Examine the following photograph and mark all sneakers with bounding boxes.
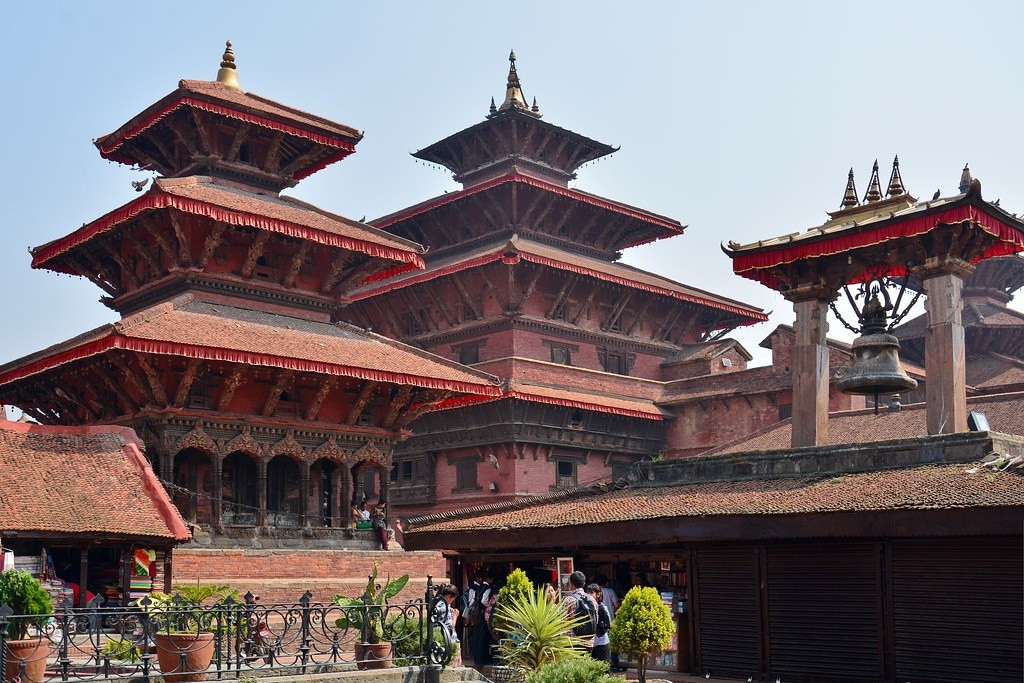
[612,666,627,672]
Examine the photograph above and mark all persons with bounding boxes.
[349,500,363,529]
[431,569,627,677]
[370,499,389,550]
[57,579,99,633]
[356,502,373,528]
[322,498,329,523]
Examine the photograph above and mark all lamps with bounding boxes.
[967,410,990,432]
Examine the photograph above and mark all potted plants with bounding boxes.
[127,591,249,683]
[332,560,412,671]
[0,566,55,682]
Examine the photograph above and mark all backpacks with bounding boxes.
[570,593,597,638]
[459,584,507,641]
